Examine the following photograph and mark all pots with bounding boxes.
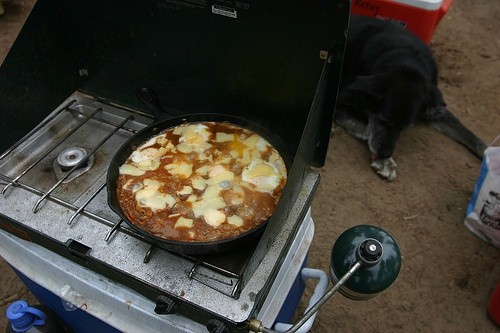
[106,114,290,253]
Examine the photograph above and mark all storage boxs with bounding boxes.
[351,0,452,47]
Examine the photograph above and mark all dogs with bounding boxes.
[333,12,488,182]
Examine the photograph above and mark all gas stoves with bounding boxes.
[0,91,322,333]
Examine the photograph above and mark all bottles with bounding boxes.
[5,300,46,333]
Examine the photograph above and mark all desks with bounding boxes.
[0,205,329,333]
